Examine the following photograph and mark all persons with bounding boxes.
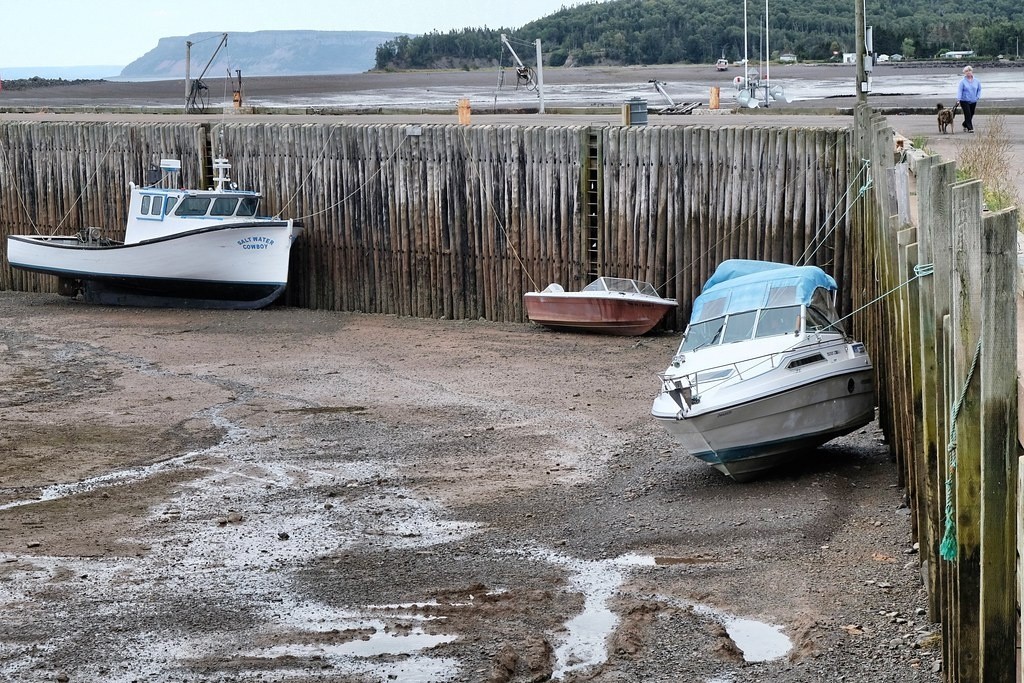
[957,66,981,132]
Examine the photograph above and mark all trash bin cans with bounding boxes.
[625,96,648,125]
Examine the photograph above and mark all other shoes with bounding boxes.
[968,130,974,132]
[962,123,967,132]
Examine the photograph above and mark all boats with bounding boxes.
[524,277,678,336]
[651,258,875,485]
[7,71,304,310]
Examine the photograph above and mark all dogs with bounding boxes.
[937,103,954,134]
